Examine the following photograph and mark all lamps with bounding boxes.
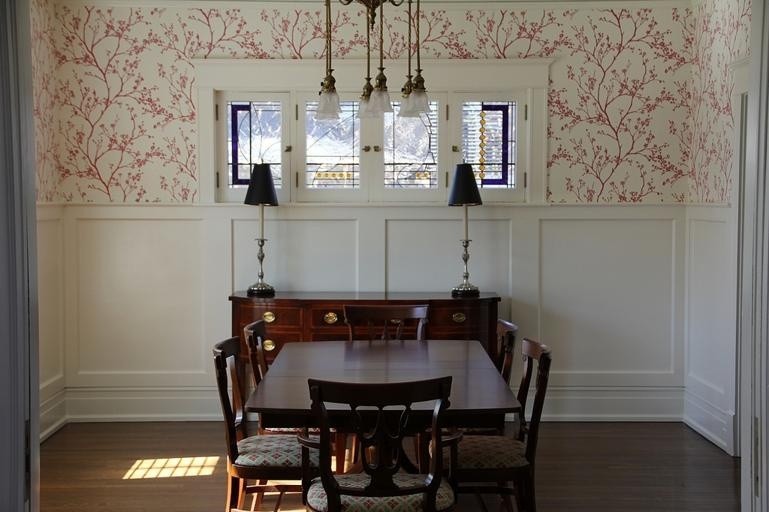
[448,162,483,297]
[315,0,432,122]
[243,163,279,296]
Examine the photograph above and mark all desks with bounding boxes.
[228,291,502,441]
[240,338,524,512]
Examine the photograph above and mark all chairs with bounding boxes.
[434,317,523,463]
[213,335,331,511]
[242,317,348,476]
[339,303,434,476]
[441,334,552,511]
[303,374,457,510]
